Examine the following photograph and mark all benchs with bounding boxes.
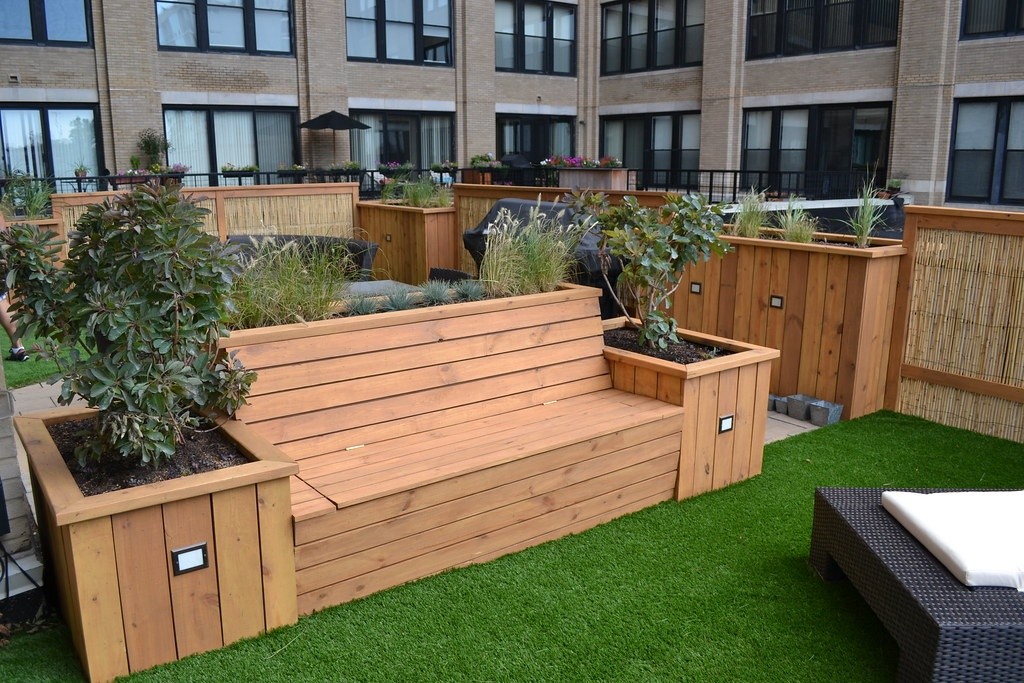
[208,289,684,620]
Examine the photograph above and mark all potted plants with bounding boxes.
[594,191,783,504]
[1,167,299,679]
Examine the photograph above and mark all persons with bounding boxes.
[0,248,30,361]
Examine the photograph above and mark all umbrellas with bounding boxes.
[295,109,372,169]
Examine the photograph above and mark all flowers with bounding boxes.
[322,152,623,170]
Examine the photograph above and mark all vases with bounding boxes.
[768,394,777,411]
[774,396,788,414]
[810,399,844,429]
[787,393,817,420]
